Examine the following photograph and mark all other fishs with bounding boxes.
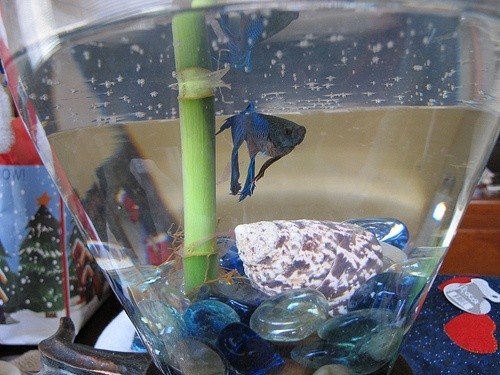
[215,103,307,202]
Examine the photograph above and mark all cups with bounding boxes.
[0,0,500,375]
